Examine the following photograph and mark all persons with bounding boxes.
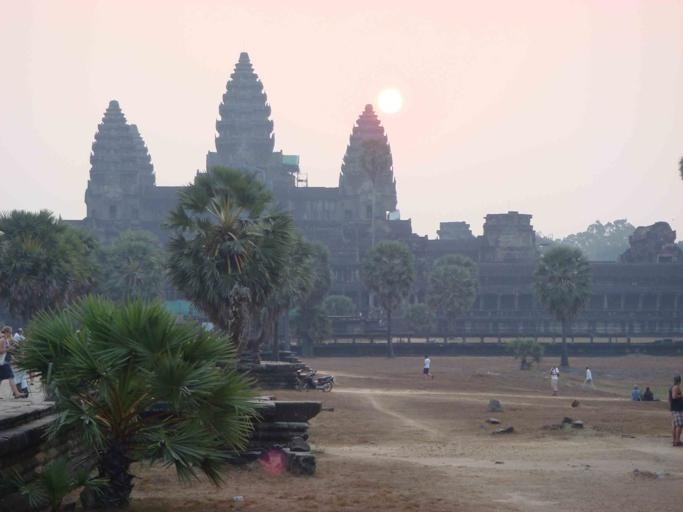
[668,374,683,446]
[631,384,640,401]
[0,326,28,398]
[582,366,593,392]
[423,354,434,381]
[642,386,654,401]
[550,364,559,396]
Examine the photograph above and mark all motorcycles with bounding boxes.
[300,367,335,393]
[293,368,308,391]
